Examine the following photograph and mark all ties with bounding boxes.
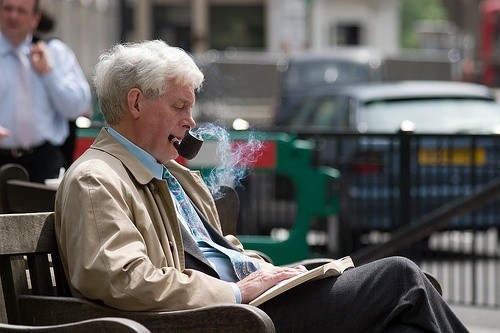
[14,47,33,149]
[163,166,258,280]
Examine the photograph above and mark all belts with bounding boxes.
[0,142,51,158]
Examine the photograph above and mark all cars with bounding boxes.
[287,83,500,261]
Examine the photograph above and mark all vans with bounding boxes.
[272,48,387,139]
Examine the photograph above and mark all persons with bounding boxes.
[54,37,471,333]
[0,0,92,269]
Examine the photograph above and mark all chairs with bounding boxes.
[0,164,443,333]
